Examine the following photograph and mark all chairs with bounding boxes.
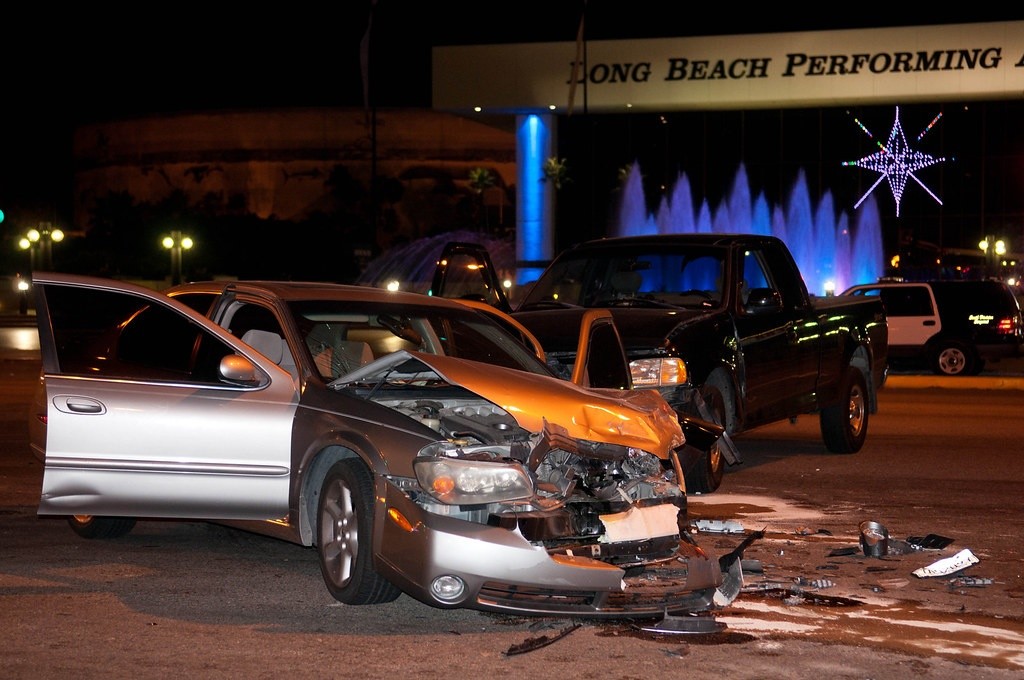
[330,341,375,378]
[715,275,745,302]
[610,272,642,298]
[235,330,283,365]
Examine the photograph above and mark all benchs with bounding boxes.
[278,338,314,381]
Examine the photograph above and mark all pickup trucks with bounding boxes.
[429,232,893,494]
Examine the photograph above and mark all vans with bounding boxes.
[828,274,1024,378]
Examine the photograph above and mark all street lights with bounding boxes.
[19,218,66,273]
[977,235,1009,277]
[162,229,197,290]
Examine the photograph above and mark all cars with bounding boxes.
[26,271,726,621]
